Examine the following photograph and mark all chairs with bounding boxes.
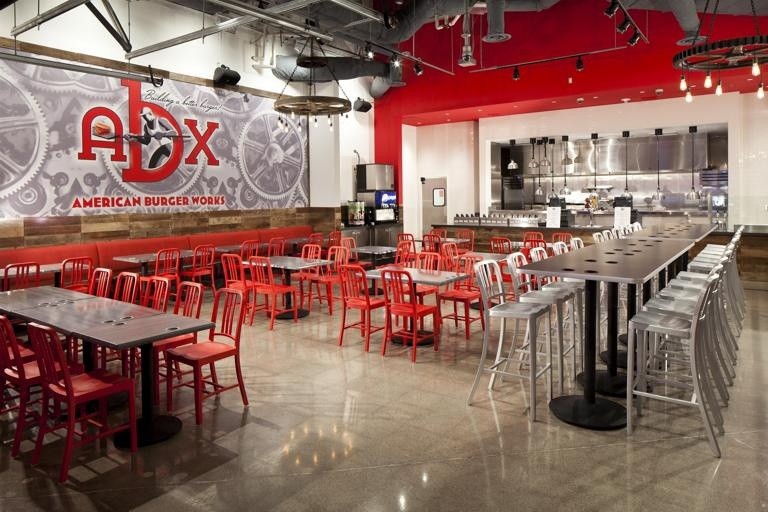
[336,229,573,363]
[113,230,353,331]
[1,257,249,487]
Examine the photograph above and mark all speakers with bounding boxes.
[214,67,240,86]
[354,100,371,112]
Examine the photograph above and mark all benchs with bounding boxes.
[1,225,317,286]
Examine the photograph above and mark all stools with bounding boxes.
[469,221,748,460]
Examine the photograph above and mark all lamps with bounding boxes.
[510,53,585,82]
[507,125,700,200]
[275,35,351,132]
[365,39,424,76]
[603,0,647,49]
[671,0,768,105]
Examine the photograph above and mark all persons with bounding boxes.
[124,104,178,169]
